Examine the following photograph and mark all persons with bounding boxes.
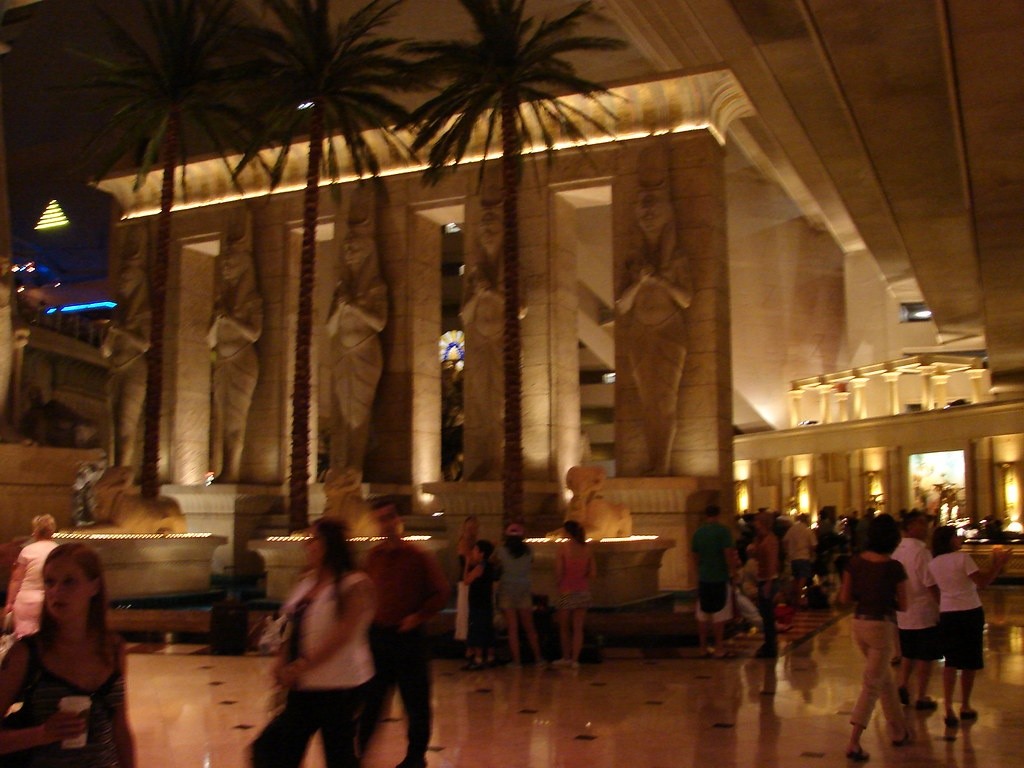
[924,525,1013,727]
[615,146,695,479]
[327,187,386,493]
[355,494,452,768]
[737,506,940,710]
[97,231,153,467]
[838,513,915,761]
[461,194,529,481]
[203,209,263,486]
[0,514,137,768]
[454,515,597,673]
[691,504,741,660]
[251,518,377,768]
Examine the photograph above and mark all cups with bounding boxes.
[58,695,92,749]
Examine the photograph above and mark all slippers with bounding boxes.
[893,730,909,745]
[847,746,870,760]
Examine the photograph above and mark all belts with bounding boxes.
[854,614,899,623]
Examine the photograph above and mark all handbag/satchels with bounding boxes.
[0,612,15,662]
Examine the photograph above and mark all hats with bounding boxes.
[506,524,524,537]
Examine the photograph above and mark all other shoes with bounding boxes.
[552,658,580,669]
[945,717,958,727]
[777,625,792,632]
[461,659,495,671]
[756,644,776,657]
[899,686,908,704]
[916,699,937,709]
[962,709,975,719]
[891,657,899,663]
